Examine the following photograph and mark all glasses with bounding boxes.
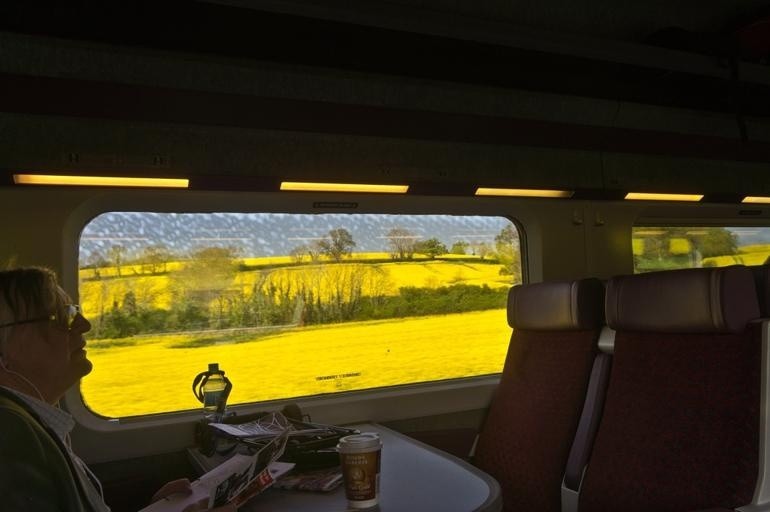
[0,304,82,332]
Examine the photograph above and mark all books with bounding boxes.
[139,453,296,511]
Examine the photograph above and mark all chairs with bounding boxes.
[561,266,769,512]
[466,276,604,512]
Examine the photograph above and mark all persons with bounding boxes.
[0,265,194,512]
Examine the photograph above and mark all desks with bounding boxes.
[187,421,504,512]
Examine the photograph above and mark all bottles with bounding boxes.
[201,362,229,432]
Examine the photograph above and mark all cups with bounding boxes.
[335,433,384,509]
